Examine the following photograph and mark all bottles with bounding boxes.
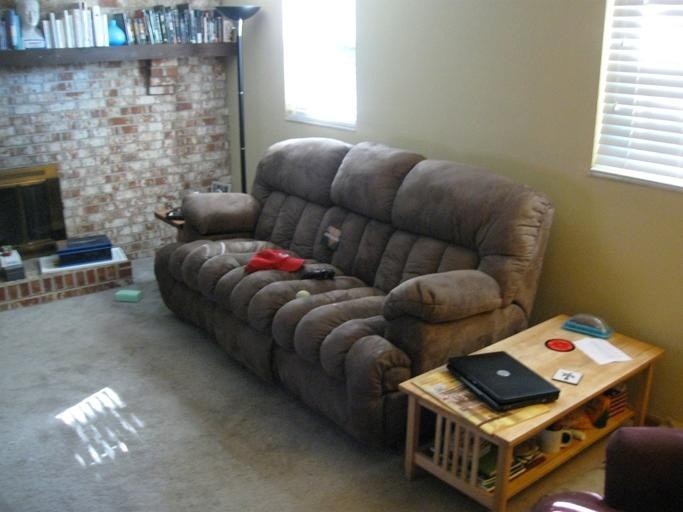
[107,19,126,45]
[5,7,21,52]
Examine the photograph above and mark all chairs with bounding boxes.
[533,426,683,512]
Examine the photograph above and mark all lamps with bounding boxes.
[216,5,261,193]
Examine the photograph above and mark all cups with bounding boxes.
[542,426,573,454]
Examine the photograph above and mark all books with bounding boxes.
[42,0,237,49]
[604,388,629,417]
[430,426,548,493]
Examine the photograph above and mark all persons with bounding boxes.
[16,0,45,49]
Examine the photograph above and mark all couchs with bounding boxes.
[153,137,556,455]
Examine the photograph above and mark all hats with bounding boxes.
[243,248,306,272]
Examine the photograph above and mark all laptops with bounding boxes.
[447,351,563,410]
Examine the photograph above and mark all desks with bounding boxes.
[153,205,184,228]
[398,313,667,510]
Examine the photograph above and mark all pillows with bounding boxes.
[382,268,502,325]
[179,192,261,236]
